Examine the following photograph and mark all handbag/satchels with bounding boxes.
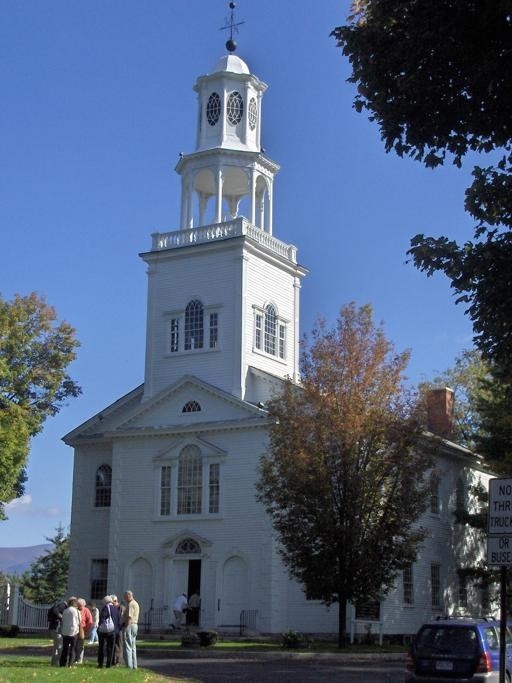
[97,604,114,633]
[79,624,84,639]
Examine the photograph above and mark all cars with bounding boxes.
[405,616,512,683]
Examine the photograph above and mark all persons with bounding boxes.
[173,590,201,628]
[47,588,139,670]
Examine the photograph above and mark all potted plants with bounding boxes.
[182,630,218,650]
[280,629,313,648]
